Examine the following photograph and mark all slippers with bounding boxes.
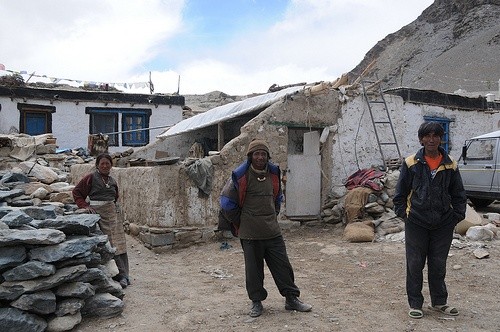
[409,308,423,318]
[429,304,459,315]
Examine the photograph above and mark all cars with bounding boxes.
[456,130,500,210]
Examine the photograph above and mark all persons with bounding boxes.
[218,140,313,317]
[393,121,467,319]
[72,154,131,286]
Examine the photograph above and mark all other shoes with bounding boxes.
[121,277,128,287]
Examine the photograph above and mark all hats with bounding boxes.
[247,140,269,153]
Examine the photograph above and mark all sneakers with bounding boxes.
[250,300,263,317]
[285,296,313,311]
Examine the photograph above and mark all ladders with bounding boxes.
[359,72,404,173]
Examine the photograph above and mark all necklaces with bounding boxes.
[102,177,110,188]
[257,177,265,181]
